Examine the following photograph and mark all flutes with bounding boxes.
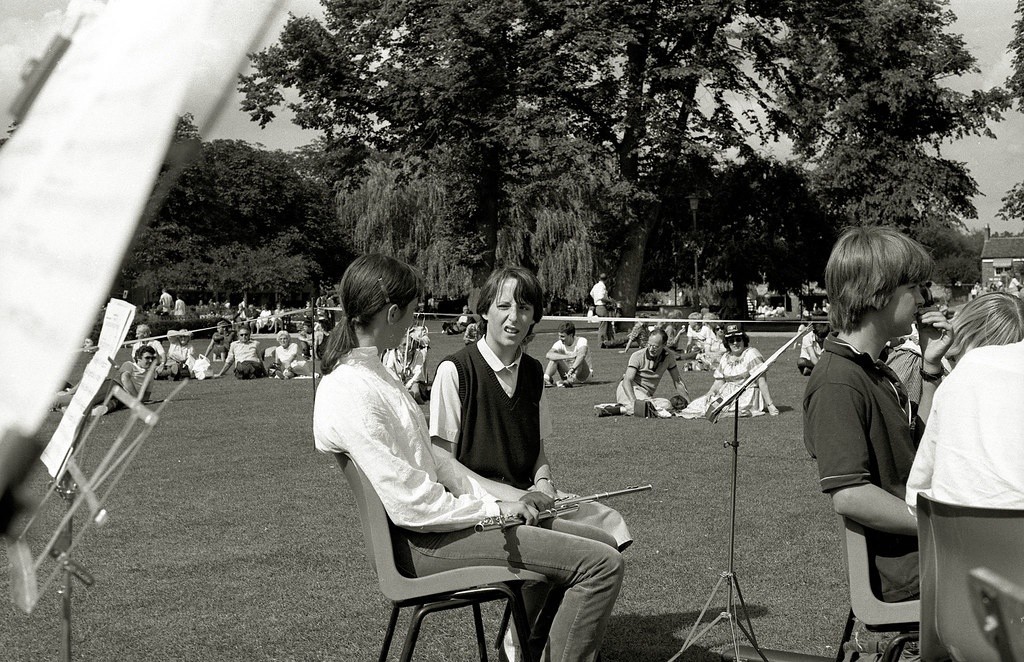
[473,483,653,532]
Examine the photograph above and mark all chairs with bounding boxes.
[835,491,1024,662]
[334,452,548,662]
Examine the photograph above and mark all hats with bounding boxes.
[723,323,743,338]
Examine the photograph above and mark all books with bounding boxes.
[40,298,137,480]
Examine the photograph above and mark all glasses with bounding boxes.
[646,344,664,350]
[144,356,157,362]
[240,333,249,337]
[727,338,743,343]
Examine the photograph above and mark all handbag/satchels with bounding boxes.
[634,399,658,419]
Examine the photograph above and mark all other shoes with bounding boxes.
[544,373,556,388]
[90,405,108,417]
[802,367,812,376]
[236,360,255,379]
[593,404,623,417]
[167,364,180,382]
[561,379,574,388]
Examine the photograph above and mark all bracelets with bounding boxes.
[536,477,554,487]
[918,365,944,382]
[768,401,773,406]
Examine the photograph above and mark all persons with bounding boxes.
[677,323,779,419]
[920,281,935,308]
[747,297,787,318]
[204,317,329,380]
[797,324,830,376]
[82,334,99,353]
[802,226,954,603]
[971,273,1024,300]
[594,329,691,418]
[49,345,156,416]
[132,324,194,380]
[314,254,632,662]
[884,292,1024,402]
[906,337,1024,526]
[603,310,725,371]
[159,287,287,335]
